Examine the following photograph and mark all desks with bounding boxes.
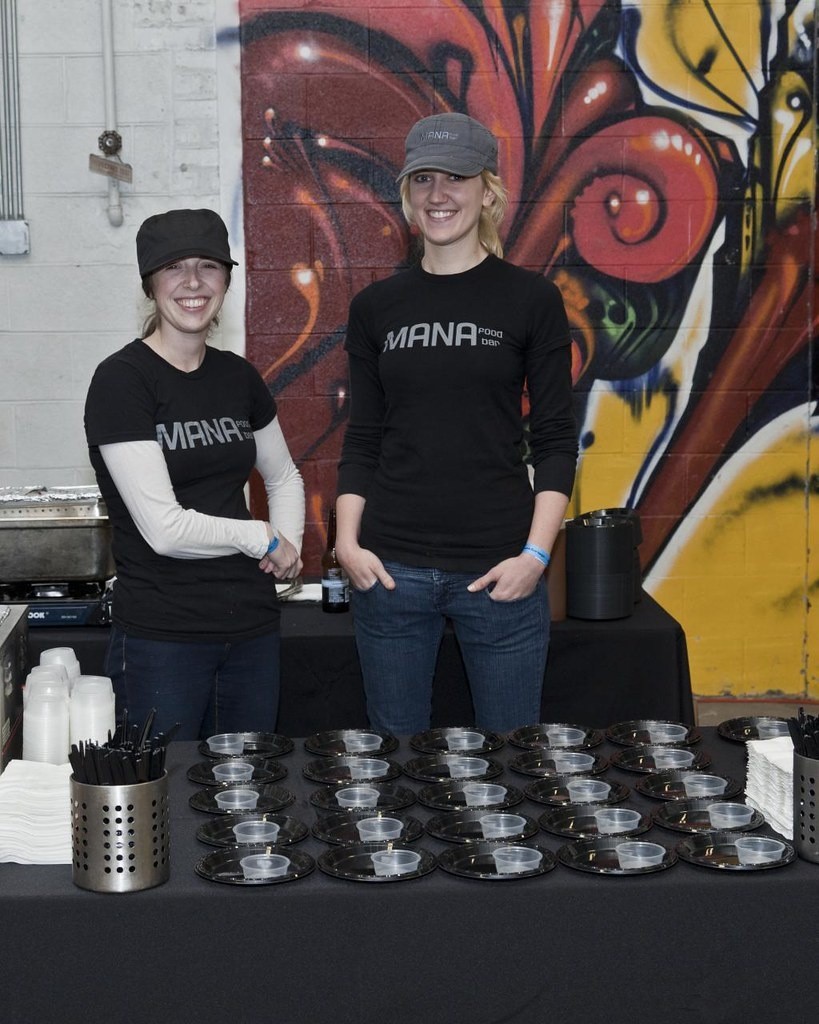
[26,575,698,742]
[1,724,819,1024]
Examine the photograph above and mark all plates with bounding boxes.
[565,507,643,621]
[186,715,797,887]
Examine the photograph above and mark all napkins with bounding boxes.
[1,759,77,865]
[743,736,795,841]
[275,583,324,602]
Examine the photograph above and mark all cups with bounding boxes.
[22,648,116,766]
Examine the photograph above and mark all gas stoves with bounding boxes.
[0,576,107,604]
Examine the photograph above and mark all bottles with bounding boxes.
[322,510,350,613]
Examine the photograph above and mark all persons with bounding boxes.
[84,208,305,741]
[333,112,579,735]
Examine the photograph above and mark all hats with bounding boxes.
[395,112,497,183]
[136,209,239,277]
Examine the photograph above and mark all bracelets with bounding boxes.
[267,525,279,554]
[522,543,550,567]
